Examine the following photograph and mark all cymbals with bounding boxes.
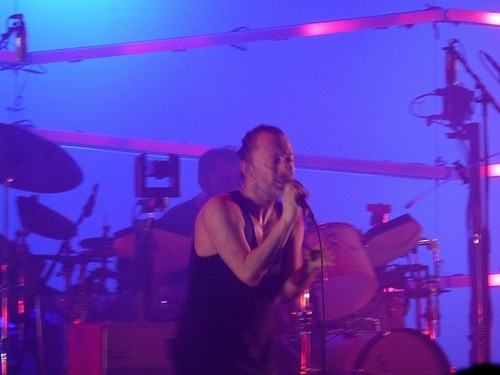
[0,120,83,194]
[17,195,78,240]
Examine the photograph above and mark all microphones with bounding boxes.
[445,52,456,85]
[295,195,314,220]
[84,184,98,216]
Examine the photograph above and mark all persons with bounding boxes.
[153,147,241,239]
[174,124,337,375]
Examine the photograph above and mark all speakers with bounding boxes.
[65,321,179,375]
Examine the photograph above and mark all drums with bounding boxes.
[312,221,379,320]
[311,326,453,375]
